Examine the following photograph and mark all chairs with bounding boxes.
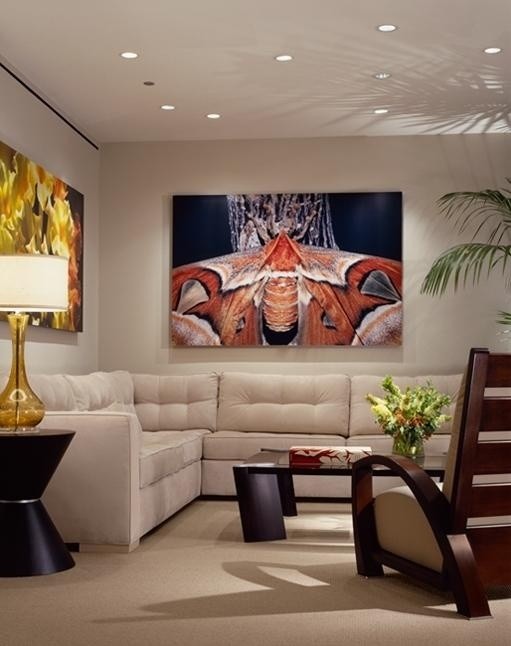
[350,348,511,620]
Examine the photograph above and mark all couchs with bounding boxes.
[201,371,511,500]
[0,369,218,553]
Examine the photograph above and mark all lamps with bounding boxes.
[0,253,70,433]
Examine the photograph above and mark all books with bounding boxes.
[288,446,373,467]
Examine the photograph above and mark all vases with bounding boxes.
[390,434,425,468]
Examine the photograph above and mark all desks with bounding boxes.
[0,429,75,577]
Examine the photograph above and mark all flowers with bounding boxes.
[365,375,453,457]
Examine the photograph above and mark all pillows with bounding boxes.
[347,373,466,435]
[218,372,349,437]
[63,370,143,435]
[131,371,219,432]
[1,374,78,415]
